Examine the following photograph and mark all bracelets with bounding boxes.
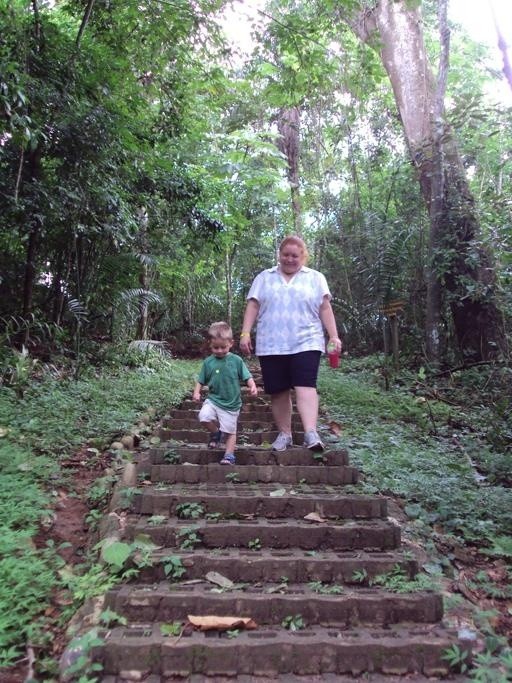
[239,332,250,338]
[330,335,338,338]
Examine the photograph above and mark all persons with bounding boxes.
[193,321,258,466]
[239,235,342,452]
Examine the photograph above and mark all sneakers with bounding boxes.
[304,431,326,450]
[271,432,293,451]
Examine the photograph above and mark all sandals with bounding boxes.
[208,431,221,448]
[220,453,236,465]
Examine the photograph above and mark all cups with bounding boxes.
[326,342,341,368]
